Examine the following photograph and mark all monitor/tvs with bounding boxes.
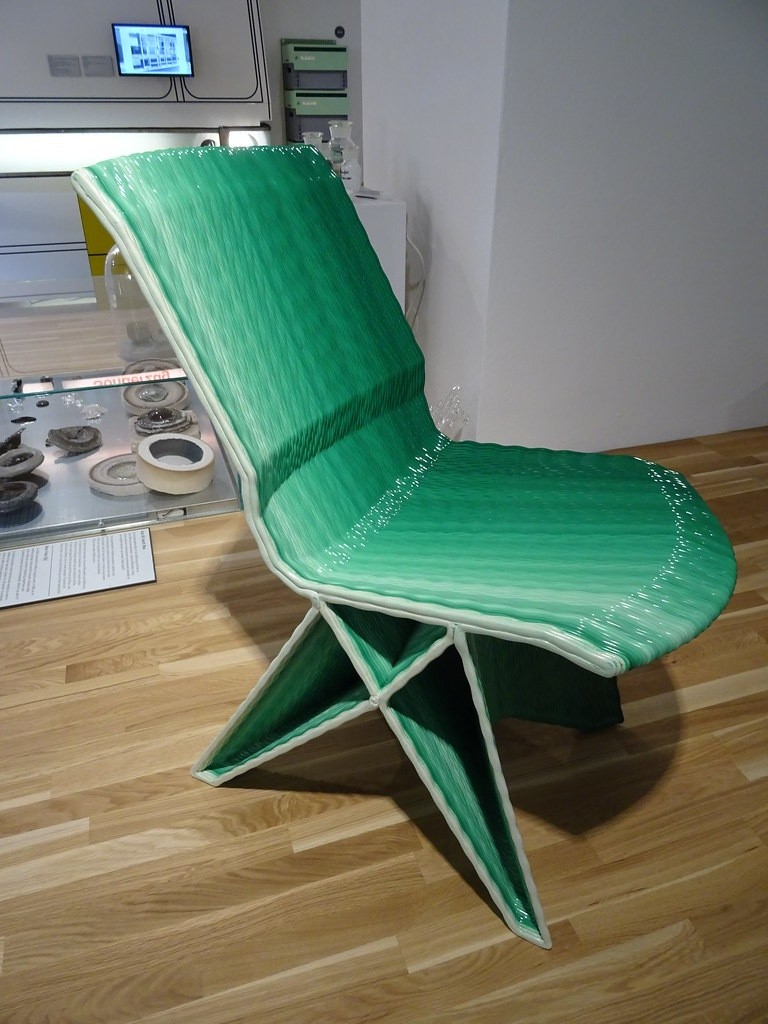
[112,23,194,78]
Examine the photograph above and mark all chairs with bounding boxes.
[72,143,737,951]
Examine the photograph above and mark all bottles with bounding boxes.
[301,130,328,162]
[327,119,363,193]
[104,243,174,361]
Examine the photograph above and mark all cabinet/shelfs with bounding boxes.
[0,126,284,549]
